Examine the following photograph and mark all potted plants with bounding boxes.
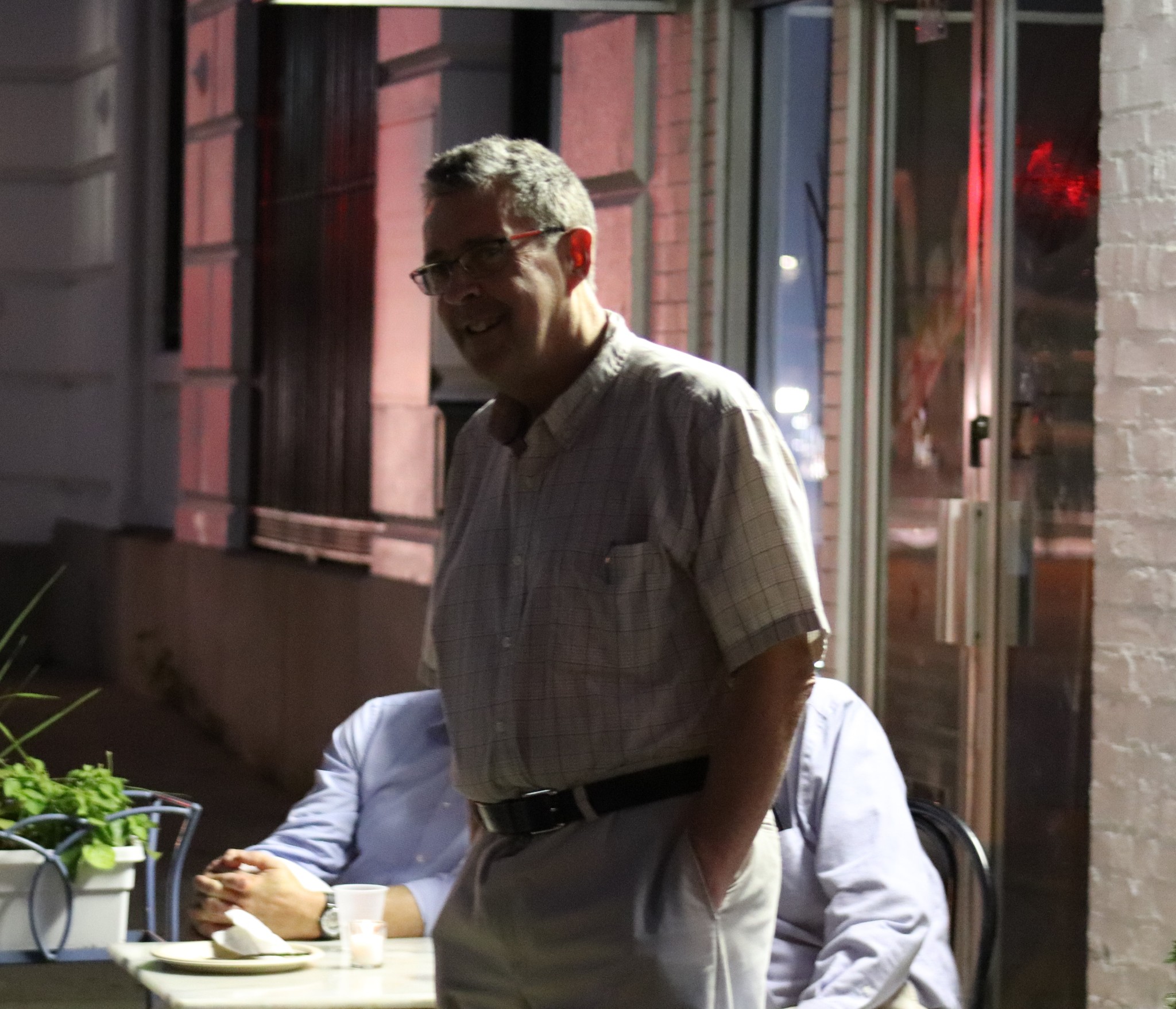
[0,757,165,950]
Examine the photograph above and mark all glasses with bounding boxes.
[409,226,570,296]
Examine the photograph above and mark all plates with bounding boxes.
[151,941,324,973]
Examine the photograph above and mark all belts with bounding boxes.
[469,755,708,834]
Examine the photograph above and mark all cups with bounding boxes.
[332,884,390,950]
[351,920,388,969]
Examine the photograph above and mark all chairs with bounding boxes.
[907,799,1001,1009]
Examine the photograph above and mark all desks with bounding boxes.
[101,920,439,1009]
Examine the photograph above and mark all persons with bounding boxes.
[771,678,967,1009]
[409,134,831,1009]
[191,690,468,942]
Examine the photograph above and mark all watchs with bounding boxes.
[320,890,348,942]
